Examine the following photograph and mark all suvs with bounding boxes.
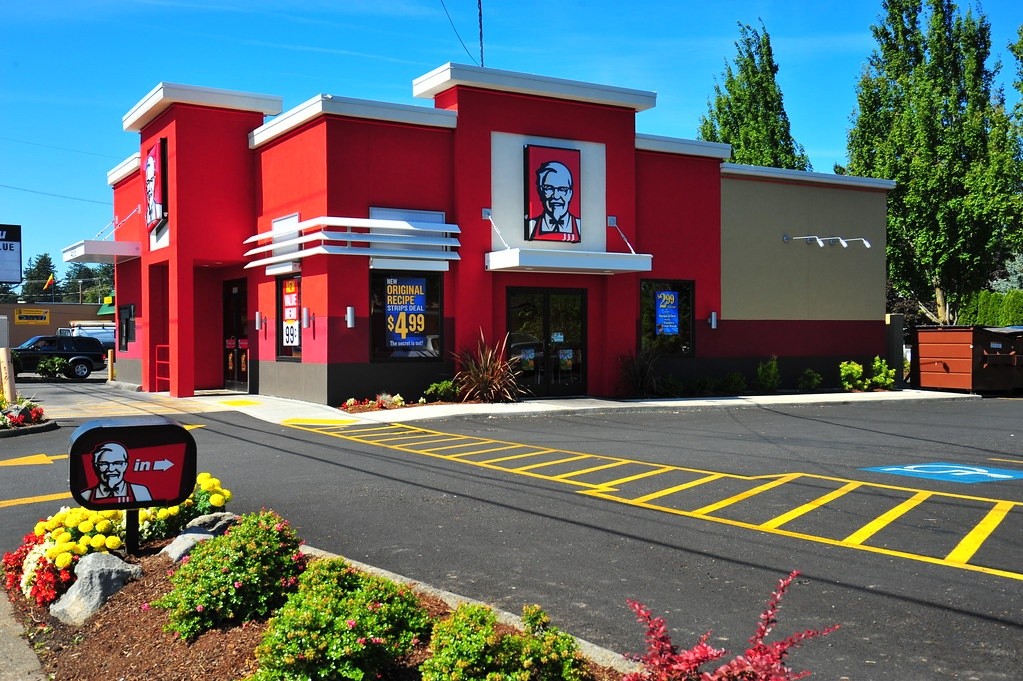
[56,320,116,361]
[10,335,107,379]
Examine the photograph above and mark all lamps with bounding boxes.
[807,237,848,249]
[303,308,315,328]
[255,312,266,330]
[782,235,823,247]
[830,237,871,249]
[346,306,356,329]
